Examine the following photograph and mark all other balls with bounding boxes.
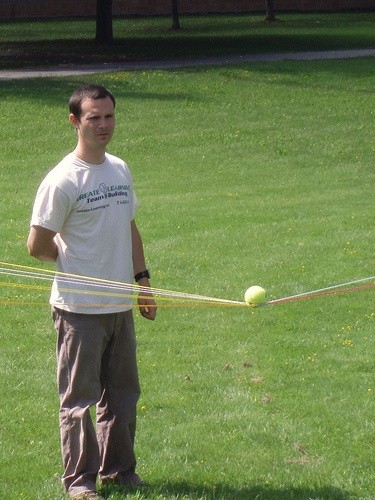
[246,285,266,305]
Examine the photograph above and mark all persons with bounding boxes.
[25,83,159,500]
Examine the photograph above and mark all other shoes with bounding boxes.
[73,490,106,500]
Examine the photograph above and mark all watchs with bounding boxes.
[132,268,152,283]
